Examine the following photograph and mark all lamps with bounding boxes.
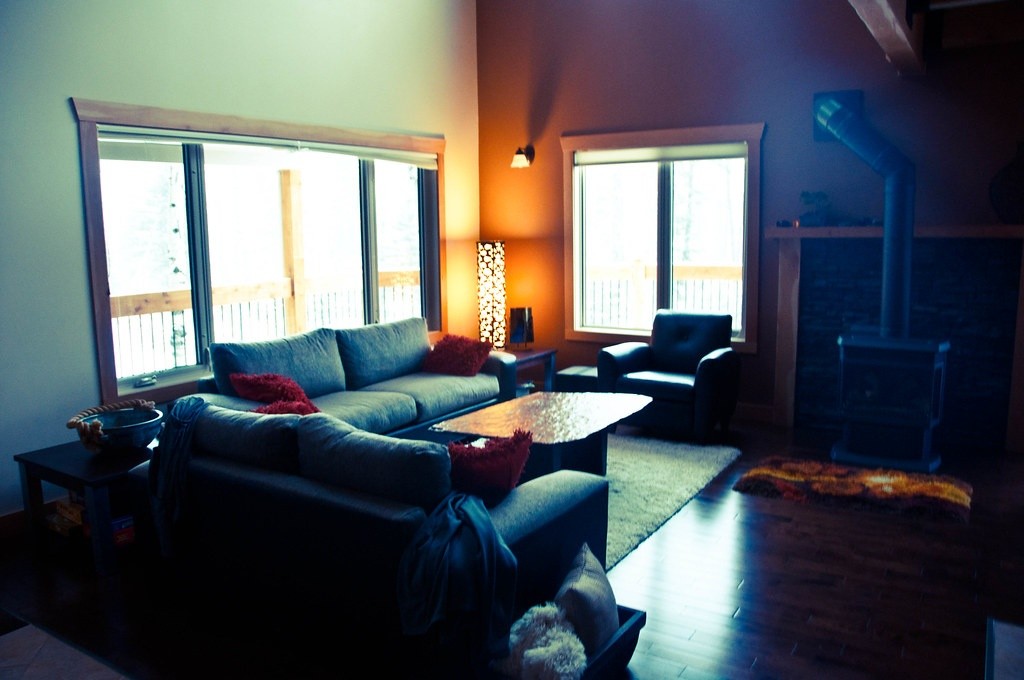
[511,146,535,168]
[509,307,534,351]
[477,239,508,351]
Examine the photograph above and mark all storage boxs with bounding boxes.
[488,605,646,680]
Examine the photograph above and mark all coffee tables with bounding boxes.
[426,391,653,487]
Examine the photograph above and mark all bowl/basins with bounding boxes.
[78,408,164,459]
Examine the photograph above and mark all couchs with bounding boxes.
[166,317,517,447]
[597,309,738,447]
[149,395,609,680]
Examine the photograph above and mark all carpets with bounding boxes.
[464,433,740,571]
[730,447,973,525]
[0,603,143,680]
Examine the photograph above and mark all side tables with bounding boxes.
[14,439,177,635]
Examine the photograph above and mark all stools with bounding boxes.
[555,365,598,392]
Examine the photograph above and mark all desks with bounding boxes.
[508,349,558,398]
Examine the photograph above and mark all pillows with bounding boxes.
[420,333,493,377]
[552,542,620,663]
[250,401,318,416]
[448,428,533,507]
[228,372,321,412]
